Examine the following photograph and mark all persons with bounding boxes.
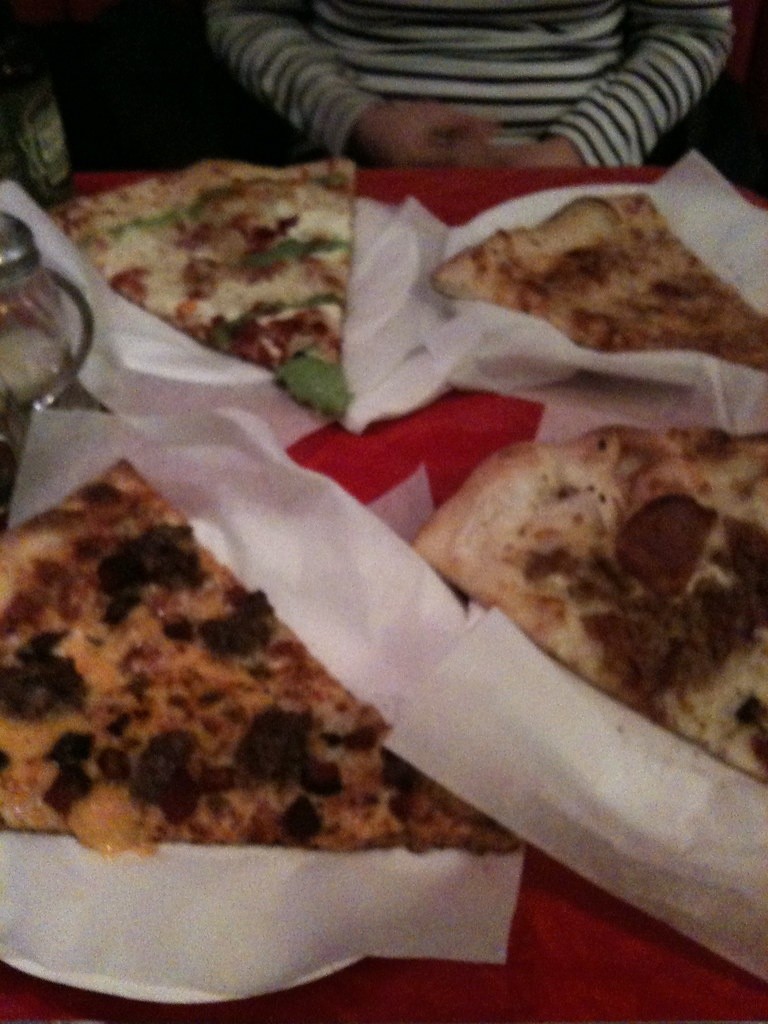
[201,1,742,173]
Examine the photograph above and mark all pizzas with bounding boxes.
[0,154,767,855]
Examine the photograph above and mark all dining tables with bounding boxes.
[1,168,766,1021]
